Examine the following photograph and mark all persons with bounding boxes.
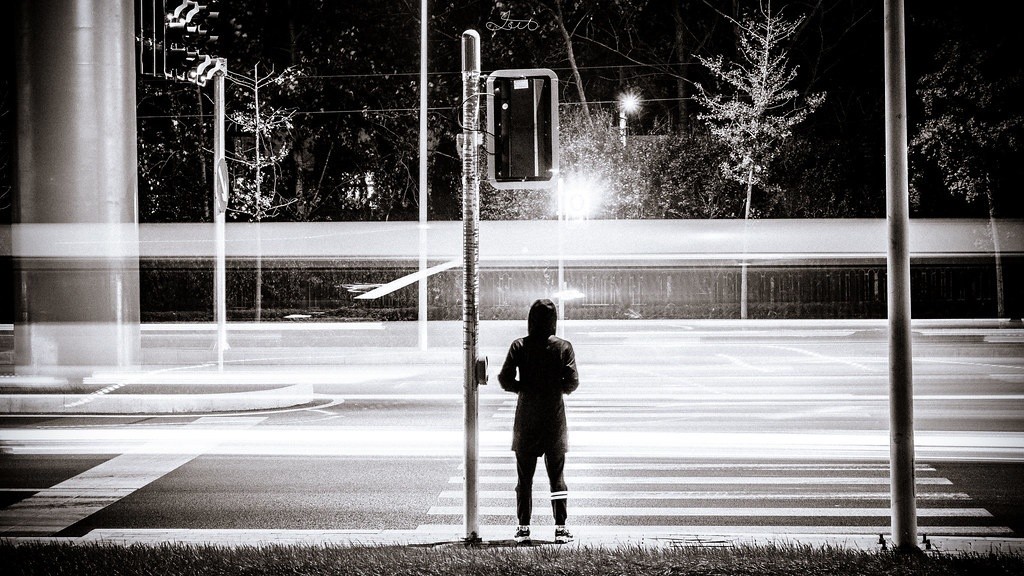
[497,299,580,544]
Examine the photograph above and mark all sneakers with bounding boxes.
[513,527,530,542]
[555,527,574,546]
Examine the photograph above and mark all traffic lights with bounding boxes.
[167,47,222,87]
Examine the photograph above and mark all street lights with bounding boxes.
[558,178,594,320]
[619,92,638,150]
